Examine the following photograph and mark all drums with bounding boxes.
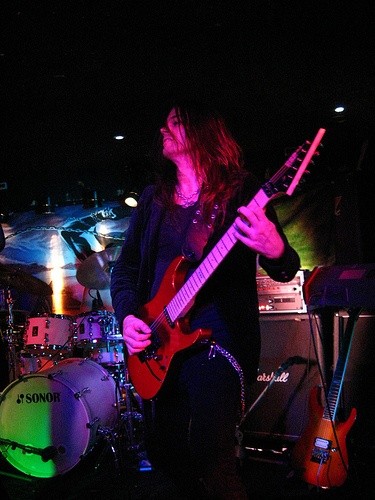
[86,349,123,371]
[73,311,123,350]
[0,359,124,479]
[23,314,74,358]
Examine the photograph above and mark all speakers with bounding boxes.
[242,309,375,468]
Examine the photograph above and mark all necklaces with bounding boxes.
[175,182,200,208]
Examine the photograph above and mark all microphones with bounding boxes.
[96,290,104,308]
[40,446,58,462]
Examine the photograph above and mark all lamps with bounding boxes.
[42,188,141,218]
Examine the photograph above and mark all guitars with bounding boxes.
[125,128,327,400]
[293,302,359,488]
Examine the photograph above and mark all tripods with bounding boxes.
[104,371,149,450]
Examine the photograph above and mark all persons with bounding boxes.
[109,97,301,500]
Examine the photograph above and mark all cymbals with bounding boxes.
[0,267,52,298]
[0,263,50,276]
[75,246,122,290]
